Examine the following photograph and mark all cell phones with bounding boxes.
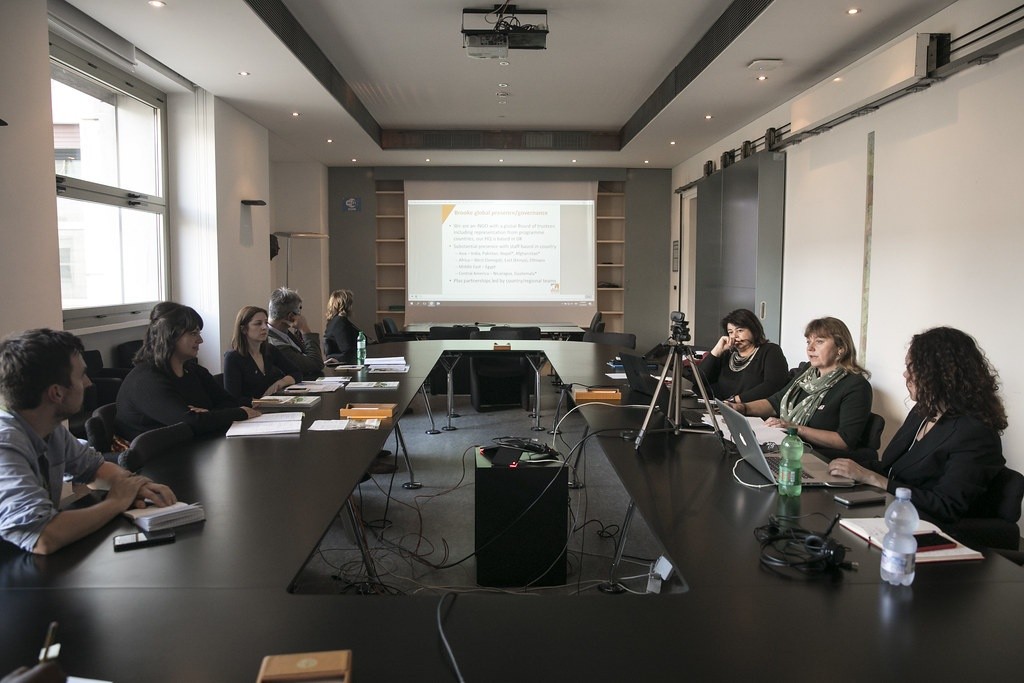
[834,490,886,505]
[285,388,309,391]
[913,532,957,552]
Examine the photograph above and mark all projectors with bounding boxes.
[463,31,545,58]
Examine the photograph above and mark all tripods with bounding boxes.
[635,333,727,456]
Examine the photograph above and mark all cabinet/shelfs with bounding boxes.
[375,178,406,330]
[596,181,628,332]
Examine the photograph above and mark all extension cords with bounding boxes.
[646,563,661,594]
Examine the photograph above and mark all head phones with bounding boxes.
[759,531,846,568]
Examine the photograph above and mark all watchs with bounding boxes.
[728,394,736,403]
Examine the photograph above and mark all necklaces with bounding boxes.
[923,421,928,435]
[730,346,759,372]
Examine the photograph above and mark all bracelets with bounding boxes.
[740,402,747,416]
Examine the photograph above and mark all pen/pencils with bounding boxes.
[126,468,142,478]
[39,621,58,663]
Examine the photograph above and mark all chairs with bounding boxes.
[68,318,407,494]
[430,313,1024,562]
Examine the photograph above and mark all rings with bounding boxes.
[777,419,780,421]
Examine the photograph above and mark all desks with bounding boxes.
[0,323,1024,683]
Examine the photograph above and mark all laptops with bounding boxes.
[619,352,855,487]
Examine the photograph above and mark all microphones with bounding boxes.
[823,513,842,537]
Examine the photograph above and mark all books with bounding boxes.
[701,413,789,447]
[345,381,399,390]
[122,495,206,531]
[608,356,658,370]
[259,395,321,407]
[837,516,984,567]
[226,411,305,437]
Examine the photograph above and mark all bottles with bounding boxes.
[880,487,919,586]
[356,331,366,359]
[778,428,804,496]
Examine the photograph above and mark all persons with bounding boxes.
[224,305,372,484]
[263,286,400,474]
[323,288,392,456]
[107,299,261,456]
[691,308,790,413]
[722,317,873,463]
[827,326,1021,556]
[0,327,175,560]
